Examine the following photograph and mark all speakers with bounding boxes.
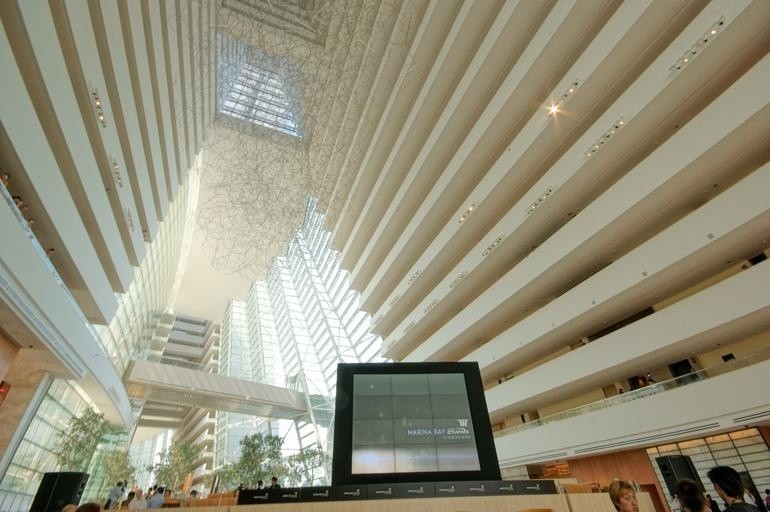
[655,455,707,498]
[29,472,90,512]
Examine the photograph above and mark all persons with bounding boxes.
[257,480,264,489]
[13,195,36,227]
[2,173,13,184]
[270,477,281,488]
[235,483,243,493]
[105,480,172,509]
[618,389,624,394]
[671,466,770,512]
[609,480,640,512]
[45,247,56,258]
[76,502,101,512]
[578,338,585,346]
[189,489,199,496]
[62,503,78,512]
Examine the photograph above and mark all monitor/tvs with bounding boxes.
[330,362,502,487]
